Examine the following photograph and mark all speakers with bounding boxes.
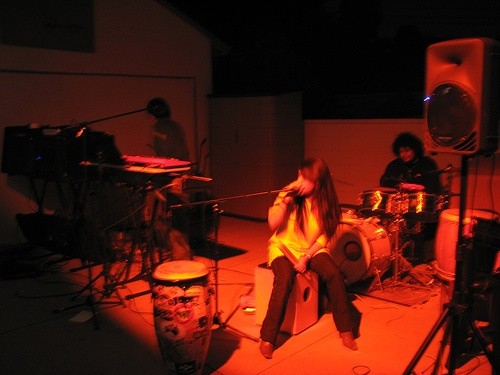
[424,36,500,156]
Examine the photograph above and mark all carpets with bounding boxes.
[122,262,336,375]
[348,281,438,308]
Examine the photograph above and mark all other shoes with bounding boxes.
[341,332,354,349]
[260,339,274,359]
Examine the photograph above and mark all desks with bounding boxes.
[81,161,191,278]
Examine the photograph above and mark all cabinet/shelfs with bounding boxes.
[206,90,304,220]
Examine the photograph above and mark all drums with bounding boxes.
[151,260,214,375]
[400,193,447,221]
[327,218,392,282]
[358,191,396,218]
[434,208,499,283]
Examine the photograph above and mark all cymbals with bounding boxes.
[365,187,399,191]
[395,183,425,191]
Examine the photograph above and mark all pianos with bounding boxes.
[0,125,214,299]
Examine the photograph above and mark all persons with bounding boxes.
[260,158,359,359]
[377,132,441,265]
[141,97,189,233]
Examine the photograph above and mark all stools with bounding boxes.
[255,264,319,334]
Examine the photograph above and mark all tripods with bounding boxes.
[64,168,164,333]
[173,188,295,342]
[399,156,500,375]
